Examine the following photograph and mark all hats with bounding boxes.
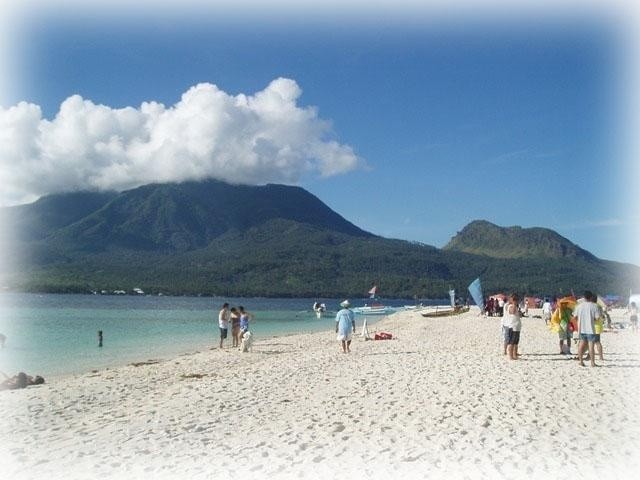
[340,299,350,308]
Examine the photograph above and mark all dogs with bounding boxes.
[240,331,253,353]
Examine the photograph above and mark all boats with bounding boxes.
[351,307,386,314]
[421,308,469,317]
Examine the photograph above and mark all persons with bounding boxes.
[237,306,252,351]
[486,291,639,367]
[335,300,356,354]
[229,307,240,348]
[218,303,230,347]
